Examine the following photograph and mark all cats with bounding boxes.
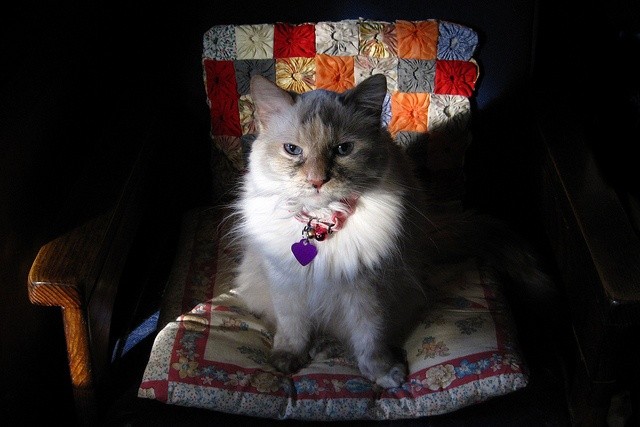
[215,72,558,391]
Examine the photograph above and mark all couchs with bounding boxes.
[28,18,640,426]
